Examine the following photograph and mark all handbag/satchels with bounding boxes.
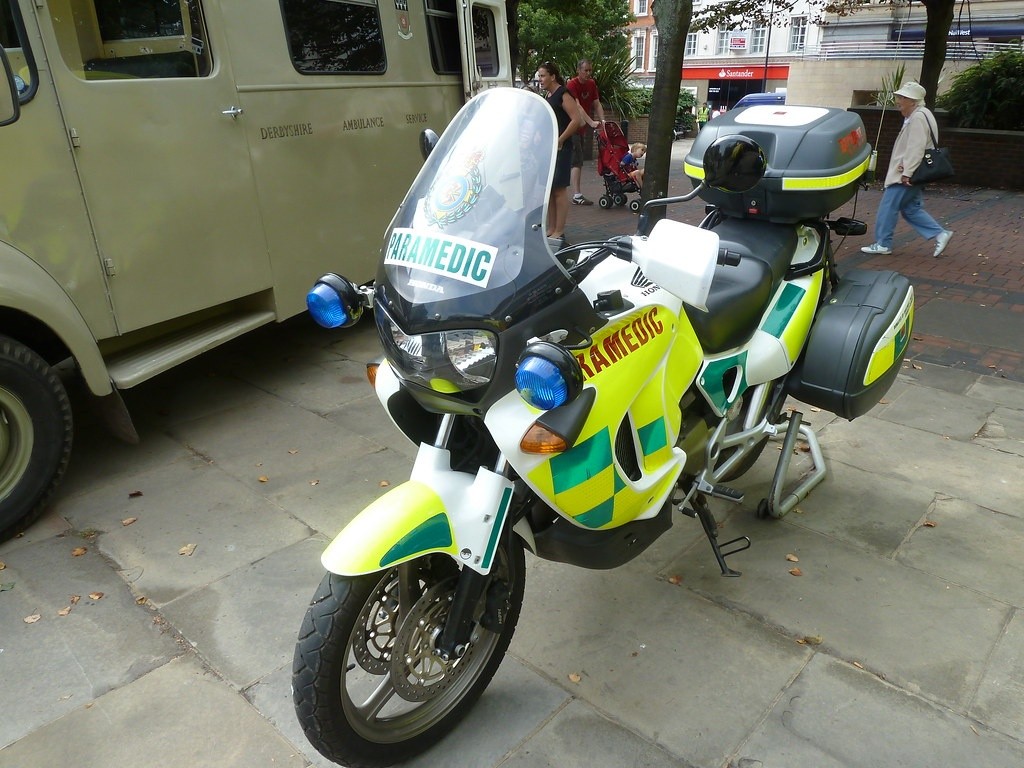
[908,111,956,184]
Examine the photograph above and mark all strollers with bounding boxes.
[594,119,642,214]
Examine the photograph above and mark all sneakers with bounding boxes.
[933,230,954,257]
[572,194,593,205]
[861,242,891,254]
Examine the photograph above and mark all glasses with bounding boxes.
[545,61,551,66]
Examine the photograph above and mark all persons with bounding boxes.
[566,58,605,205]
[860,82,954,256]
[516,62,582,239]
[619,142,647,189]
[696,102,710,131]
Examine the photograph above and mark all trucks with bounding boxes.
[0,0,521,547]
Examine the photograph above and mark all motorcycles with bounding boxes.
[289,83,916,767]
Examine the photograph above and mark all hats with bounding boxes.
[893,81,926,99]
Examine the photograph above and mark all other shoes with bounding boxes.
[547,230,565,240]
